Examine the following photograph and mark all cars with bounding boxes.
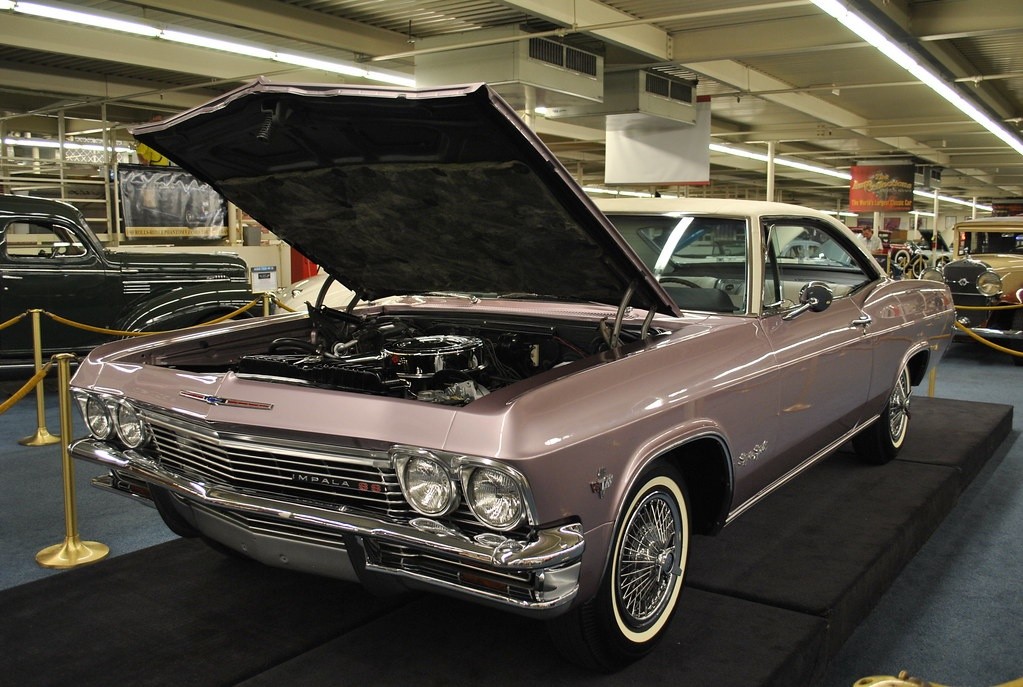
[0,193,276,383]
[777,228,954,282]
[68,71,961,669]
[941,214,1023,365]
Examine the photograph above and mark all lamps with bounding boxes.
[0,0,416,88]
[812,0,1023,156]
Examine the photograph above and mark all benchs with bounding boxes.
[663,276,849,310]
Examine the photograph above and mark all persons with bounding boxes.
[858,225,883,251]
